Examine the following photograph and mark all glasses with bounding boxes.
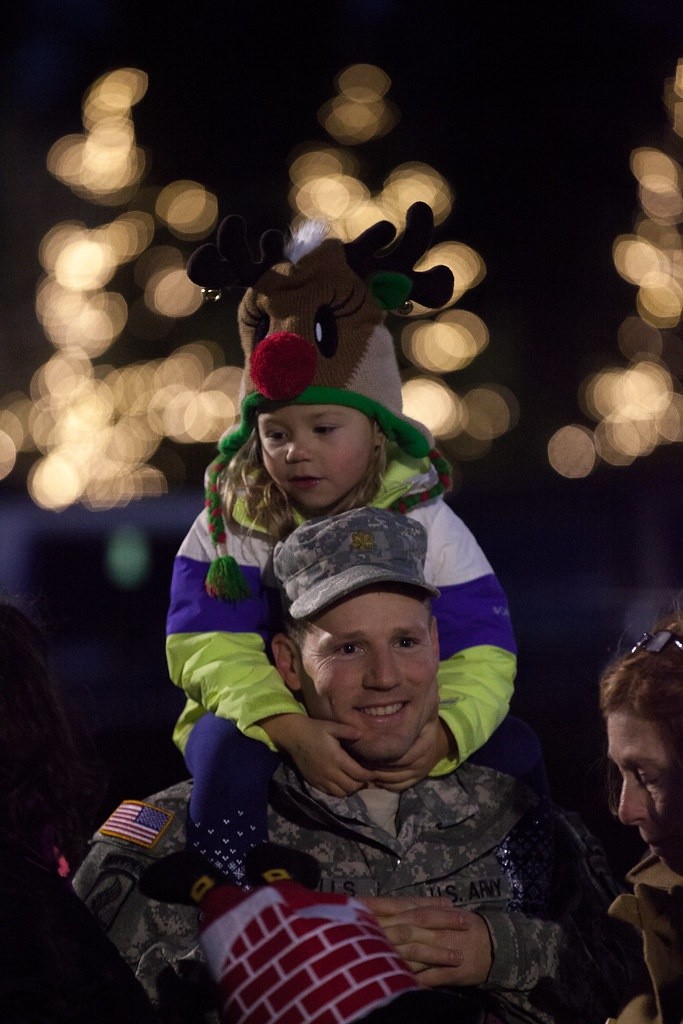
[626,631,683,660]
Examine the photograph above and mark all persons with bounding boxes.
[165,404,517,892]
[73,505,652,1024]
[599,620,683,1024]
[0,602,157,1024]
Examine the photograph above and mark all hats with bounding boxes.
[269,507,441,620]
[184,200,456,598]
